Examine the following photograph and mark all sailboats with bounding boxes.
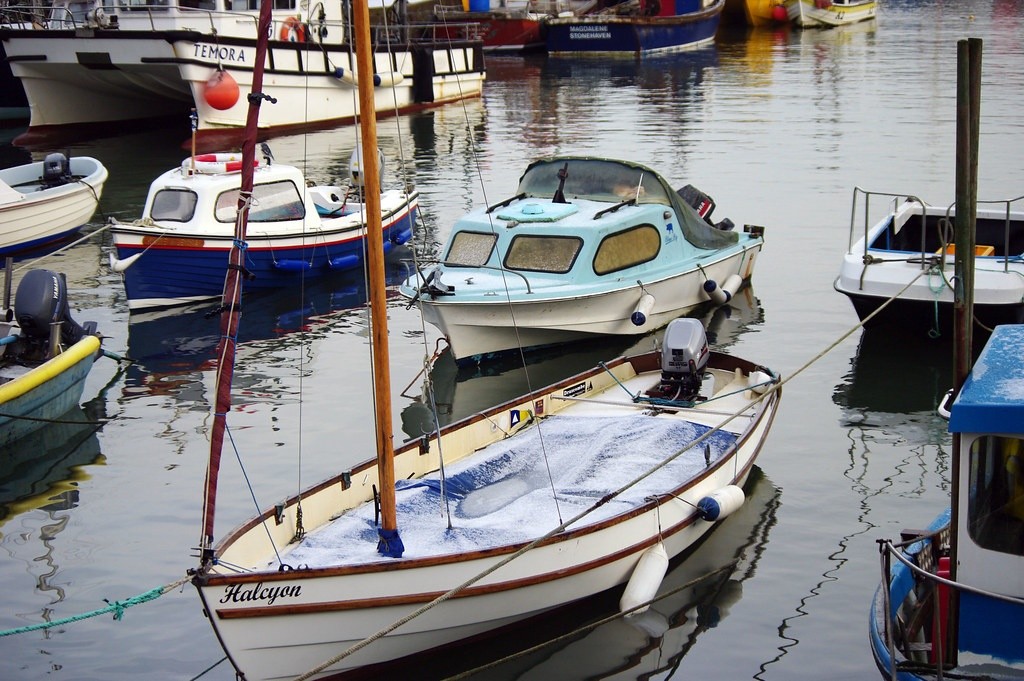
[172,0,785,681]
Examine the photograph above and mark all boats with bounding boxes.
[0,0,880,153]
[831,185,1024,347]
[97,108,421,316]
[0,150,110,259]
[393,153,766,373]
[0,256,116,454]
[869,323,1024,680]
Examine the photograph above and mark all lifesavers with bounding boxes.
[280,16,305,44]
[181,152,259,176]
